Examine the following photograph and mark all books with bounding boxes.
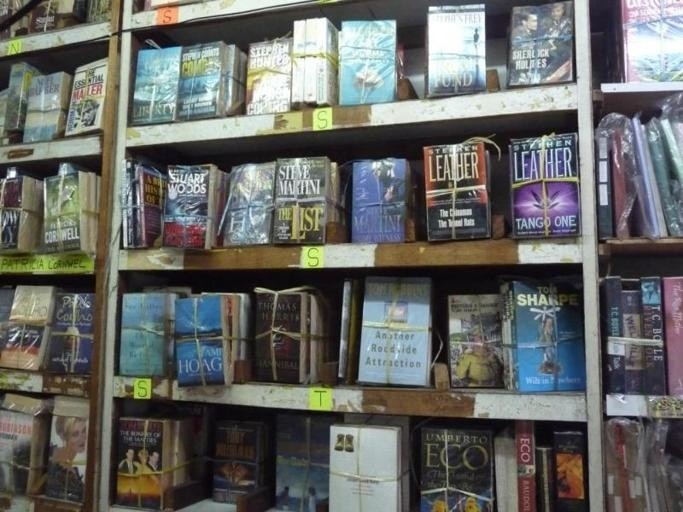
[293,18,338,106]
[602,275,682,394]
[603,418,683,512]
[0,55,114,148]
[115,284,249,386]
[424,139,493,242]
[448,276,586,392]
[131,42,246,128]
[0,386,90,507]
[352,156,417,242]
[0,0,223,44]
[610,0,682,83]
[255,286,328,387]
[508,0,577,88]
[330,413,421,511]
[0,163,100,256]
[242,38,291,117]
[336,19,398,104]
[507,133,581,240]
[274,410,329,511]
[110,416,211,512]
[595,114,682,240]
[427,4,485,98]
[119,155,349,247]
[0,285,96,378]
[337,275,433,388]
[214,419,260,503]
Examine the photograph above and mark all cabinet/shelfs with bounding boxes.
[98,0,683,511]
[0,1,121,512]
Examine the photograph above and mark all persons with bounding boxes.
[46,416,87,504]
[276,486,318,511]
[117,447,160,489]
[424,421,592,511]
[512,4,571,83]
